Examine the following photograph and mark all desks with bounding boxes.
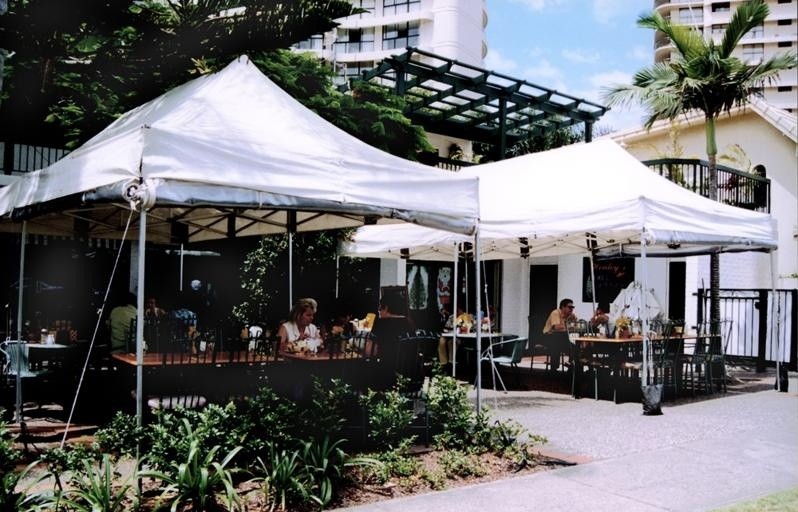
[129,350,362,367]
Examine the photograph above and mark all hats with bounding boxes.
[599,300,610,313]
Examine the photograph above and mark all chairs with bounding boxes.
[528,312,566,373]
[128,334,282,350]
[472,338,529,396]
[0,340,45,389]
[565,316,733,403]
[376,329,440,396]
[217,335,282,408]
[129,331,216,410]
[329,332,375,396]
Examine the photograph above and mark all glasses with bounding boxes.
[569,306,575,308]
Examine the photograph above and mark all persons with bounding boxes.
[543,300,618,375]
